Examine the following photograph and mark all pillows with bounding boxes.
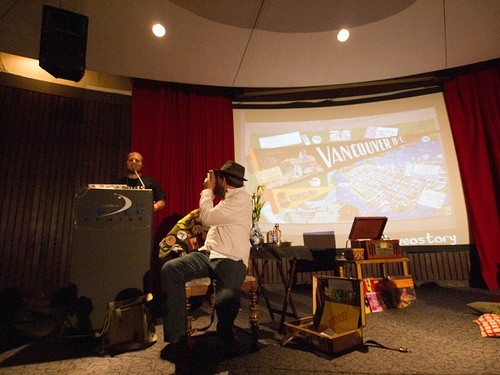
[466,301,500,337]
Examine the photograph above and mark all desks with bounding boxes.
[337,257,409,279]
[251,245,314,334]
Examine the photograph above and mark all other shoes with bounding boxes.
[174,348,195,375]
[222,327,243,356]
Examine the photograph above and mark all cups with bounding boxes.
[267,231,273,243]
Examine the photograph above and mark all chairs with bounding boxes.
[159,208,261,345]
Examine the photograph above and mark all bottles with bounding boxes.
[273,224,281,247]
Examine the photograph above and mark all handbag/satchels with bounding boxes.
[94,293,158,358]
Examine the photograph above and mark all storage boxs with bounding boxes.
[284,275,366,354]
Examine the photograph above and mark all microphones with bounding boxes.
[135,170,145,189]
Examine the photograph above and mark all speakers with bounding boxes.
[39,4,88,82]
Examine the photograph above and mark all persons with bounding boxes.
[116,152,166,213]
[399,287,414,308]
[159,159,252,360]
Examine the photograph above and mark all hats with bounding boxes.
[213,160,248,182]
